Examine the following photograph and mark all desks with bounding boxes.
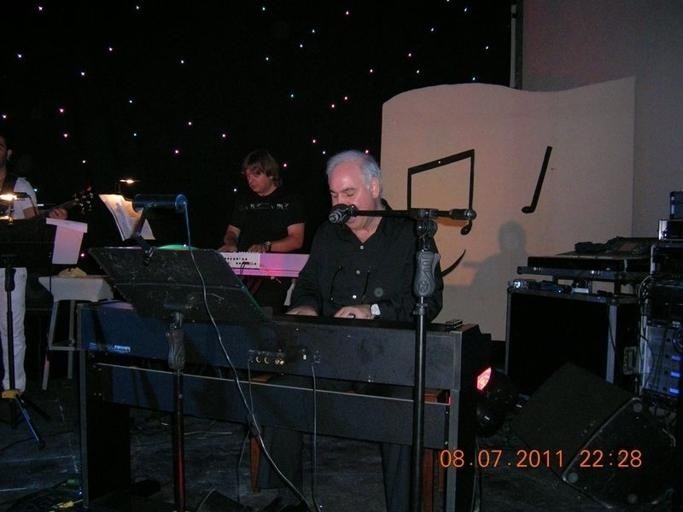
[36,275,115,391]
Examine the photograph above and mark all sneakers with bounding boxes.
[259,495,312,512]
[0,387,25,402]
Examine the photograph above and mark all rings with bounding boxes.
[348,313,355,318]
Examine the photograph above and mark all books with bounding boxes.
[97,194,156,241]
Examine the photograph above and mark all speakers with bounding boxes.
[511,361,683,512]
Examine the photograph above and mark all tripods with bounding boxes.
[0,269,50,450]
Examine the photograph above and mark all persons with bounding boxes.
[264,150,446,511]
[216,148,310,257]
[0,140,69,400]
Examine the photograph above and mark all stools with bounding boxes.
[249,372,449,512]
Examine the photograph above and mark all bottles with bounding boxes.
[509,280,529,289]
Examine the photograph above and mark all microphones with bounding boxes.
[329,203,351,224]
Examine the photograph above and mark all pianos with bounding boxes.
[74,300,492,512]
[218,252,311,279]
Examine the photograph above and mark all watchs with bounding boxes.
[264,240,271,253]
